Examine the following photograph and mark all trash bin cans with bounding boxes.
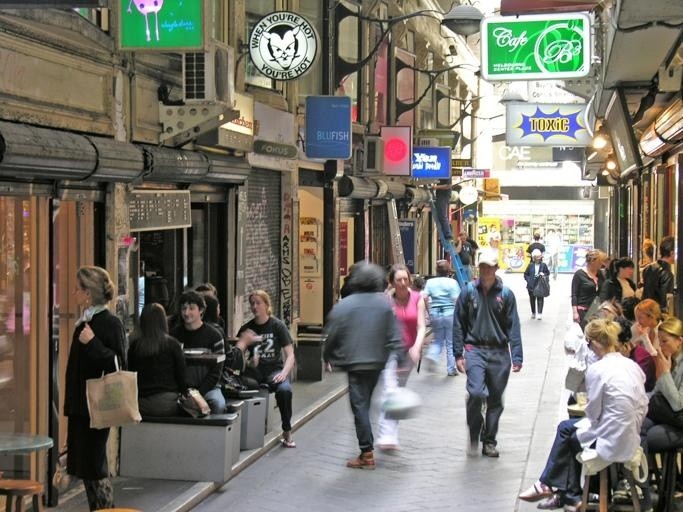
[297,336,328,382]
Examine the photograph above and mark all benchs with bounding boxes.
[258,386,275,435]
[119,414,236,482]
[241,397,266,451]
[226,400,243,465]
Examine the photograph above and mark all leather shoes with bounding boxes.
[519,482,552,502]
[537,492,583,511]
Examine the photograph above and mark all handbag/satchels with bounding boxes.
[647,391,683,431]
[564,340,587,392]
[178,386,212,419]
[532,275,551,298]
[84,353,142,431]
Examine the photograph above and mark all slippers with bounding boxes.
[279,437,297,449]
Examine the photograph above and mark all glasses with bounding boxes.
[587,339,592,348]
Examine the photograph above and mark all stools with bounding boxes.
[579,450,654,512]
[0,480,45,512]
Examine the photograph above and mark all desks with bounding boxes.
[0,432,54,456]
[185,353,226,365]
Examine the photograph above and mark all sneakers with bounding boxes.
[347,452,376,469]
[481,443,499,458]
[612,479,645,504]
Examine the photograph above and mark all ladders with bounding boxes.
[427,199,468,288]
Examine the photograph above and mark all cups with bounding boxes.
[577,391,587,405]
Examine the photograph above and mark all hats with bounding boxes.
[477,251,498,267]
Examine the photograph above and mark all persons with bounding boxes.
[488,225,501,242]
[622,296,639,320]
[641,236,675,312]
[466,235,479,266]
[432,177,453,242]
[571,249,607,334]
[548,229,559,279]
[612,318,683,512]
[524,249,550,320]
[236,290,296,448]
[410,277,431,308]
[518,318,649,509]
[600,257,636,303]
[557,317,655,512]
[454,230,472,283]
[125,302,186,416]
[63,266,127,512]
[195,282,225,328]
[203,293,256,389]
[635,239,654,299]
[322,260,399,470]
[526,234,545,260]
[630,299,662,392]
[424,259,462,376]
[170,291,226,414]
[377,264,426,451]
[452,250,523,457]
